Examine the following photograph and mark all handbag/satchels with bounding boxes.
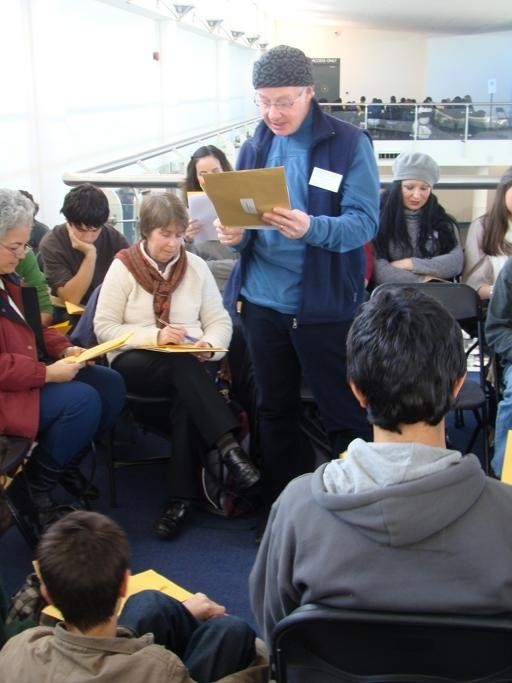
[26,497,91,542]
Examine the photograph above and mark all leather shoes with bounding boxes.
[151,501,190,540]
[220,445,262,492]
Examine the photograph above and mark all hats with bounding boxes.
[251,44,316,89]
[391,150,441,189]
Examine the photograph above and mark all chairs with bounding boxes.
[0,434,40,551]
[268,603,512,683]
[249,326,316,460]
[104,393,175,508]
[370,283,488,477]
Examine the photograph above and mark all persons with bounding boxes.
[91,190,261,541]
[212,42,380,543]
[1,188,129,553]
[459,162,512,342]
[11,188,56,327]
[1,509,270,682]
[482,250,510,484]
[37,183,132,368]
[367,149,466,287]
[249,285,510,639]
[316,93,487,137]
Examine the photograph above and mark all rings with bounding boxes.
[280,224,284,230]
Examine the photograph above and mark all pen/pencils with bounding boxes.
[157,317,198,344]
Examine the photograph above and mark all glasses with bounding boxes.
[251,86,311,108]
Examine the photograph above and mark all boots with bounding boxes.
[61,448,101,498]
[10,459,68,515]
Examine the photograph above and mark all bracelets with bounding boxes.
[488,284,495,300]
